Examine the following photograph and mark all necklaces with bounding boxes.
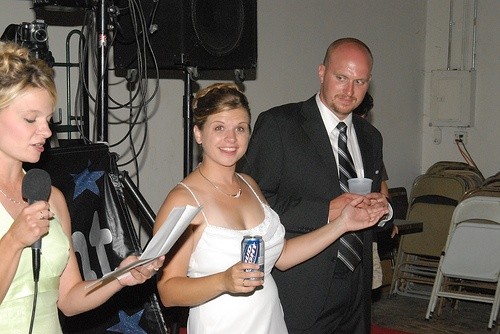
[0,190,26,204]
[196,162,243,198]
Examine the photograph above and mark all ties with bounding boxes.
[336,122,363,272]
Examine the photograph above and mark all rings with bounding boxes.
[243,280,244,286]
[154,268,159,271]
[40,211,43,220]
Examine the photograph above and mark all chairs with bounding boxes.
[388,161,500,331]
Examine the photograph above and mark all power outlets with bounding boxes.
[453,132,466,144]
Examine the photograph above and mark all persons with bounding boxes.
[237,38,399,334]
[0,41,165,334]
[153,83,389,334]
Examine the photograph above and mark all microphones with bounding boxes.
[21,169,51,281]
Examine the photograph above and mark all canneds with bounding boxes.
[241,235,265,282]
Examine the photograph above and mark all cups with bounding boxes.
[347,178,373,195]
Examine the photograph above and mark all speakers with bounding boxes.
[114,0,257,81]
